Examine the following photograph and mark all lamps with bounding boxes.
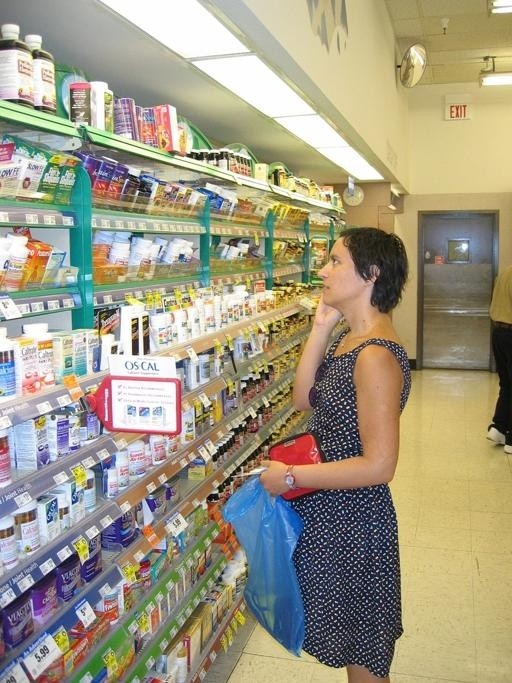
[487,0,512,17]
[479,56,512,87]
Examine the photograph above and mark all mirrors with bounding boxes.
[398,42,428,88]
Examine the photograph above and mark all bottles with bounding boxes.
[190,148,253,178]
[271,165,285,189]
[207,399,273,503]
[226,362,275,411]
[150,285,274,392]
[118,164,153,214]
[0,322,56,405]
[0,232,29,293]
[22,33,57,116]
[0,22,35,110]
[0,435,178,577]
[92,230,250,284]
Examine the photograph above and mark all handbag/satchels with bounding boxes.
[222,476,305,659]
[267,432,322,501]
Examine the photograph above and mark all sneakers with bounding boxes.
[486,421,512,454]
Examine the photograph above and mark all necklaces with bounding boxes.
[338,326,355,349]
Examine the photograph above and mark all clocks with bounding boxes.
[343,186,364,207]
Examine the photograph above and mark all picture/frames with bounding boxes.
[444,237,472,264]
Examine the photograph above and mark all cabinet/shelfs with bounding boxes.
[0,101,349,683]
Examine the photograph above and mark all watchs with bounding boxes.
[285,463,297,490]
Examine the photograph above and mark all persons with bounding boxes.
[259,227,412,683]
[485,263,512,454]
